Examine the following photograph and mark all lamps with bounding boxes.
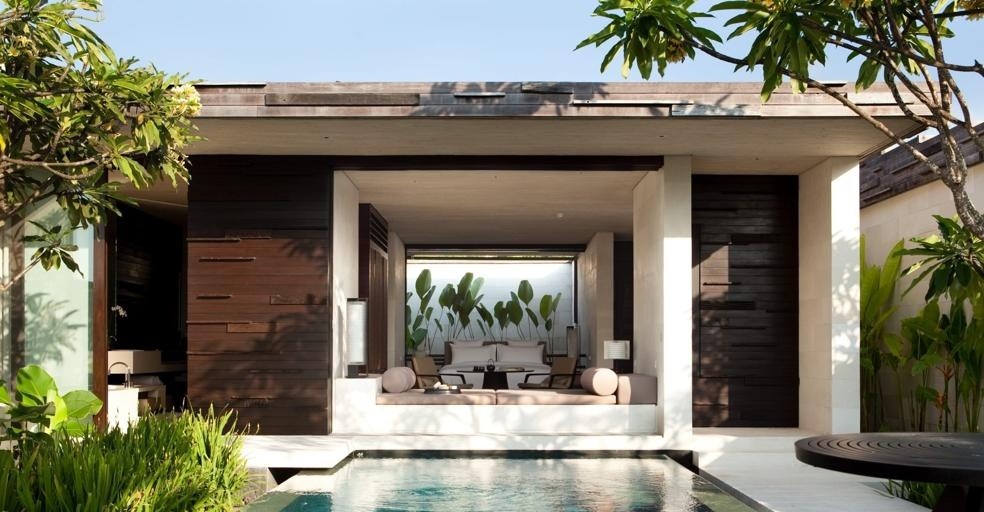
[345,298,367,378]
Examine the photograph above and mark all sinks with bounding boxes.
[107,384,125,390]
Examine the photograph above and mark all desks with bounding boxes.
[794,432,984,512]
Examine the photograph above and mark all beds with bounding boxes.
[437,340,551,390]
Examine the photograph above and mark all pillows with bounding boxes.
[382,366,416,394]
[448,343,544,363]
[579,366,619,395]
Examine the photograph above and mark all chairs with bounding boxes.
[517,356,578,390]
[410,355,473,389]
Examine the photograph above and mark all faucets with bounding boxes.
[108,361,133,387]
